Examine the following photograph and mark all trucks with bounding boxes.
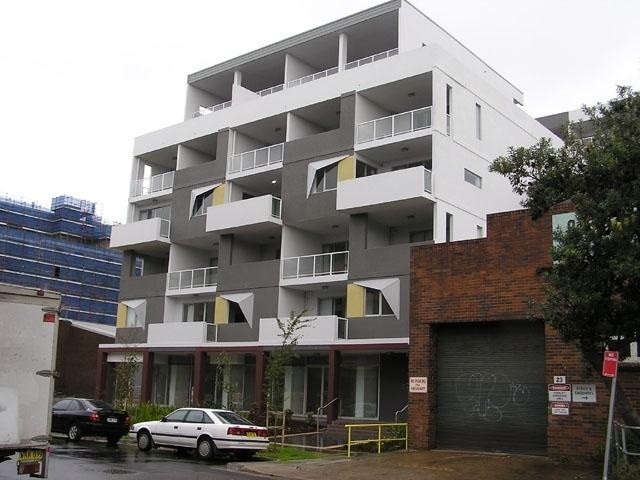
[0,283,62,480]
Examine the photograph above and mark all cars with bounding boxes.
[127,405,270,462]
[51,396,131,446]
